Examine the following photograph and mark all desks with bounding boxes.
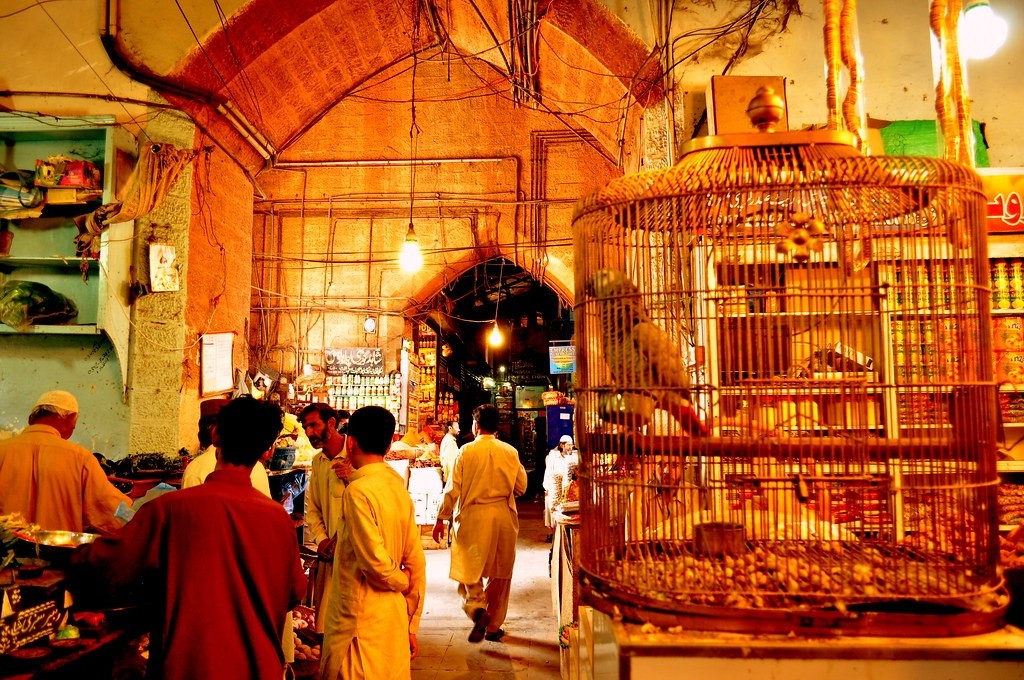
[0,588,153,679]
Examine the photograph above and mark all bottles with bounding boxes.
[418,337,435,348]
[420,366,435,384]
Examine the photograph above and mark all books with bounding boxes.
[0,179,43,211]
[834,342,873,371]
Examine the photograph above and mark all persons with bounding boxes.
[70,394,356,680]
[0,390,133,534]
[627,456,703,541]
[439,420,461,488]
[432,404,528,642]
[318,406,426,680]
[542,435,578,543]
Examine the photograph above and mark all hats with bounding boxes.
[560,434,573,443]
[202,399,227,416]
[29,390,78,425]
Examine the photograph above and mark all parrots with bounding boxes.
[581,267,711,439]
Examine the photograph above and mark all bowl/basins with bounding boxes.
[22,532,102,562]
[265,447,295,470]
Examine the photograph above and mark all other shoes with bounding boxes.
[468,608,491,643]
[485,629,504,641]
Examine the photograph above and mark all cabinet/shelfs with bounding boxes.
[547,229,1024,680]
[0,111,139,397]
[324,358,515,442]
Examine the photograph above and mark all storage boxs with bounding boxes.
[547,405,573,451]
[703,73,789,133]
[384,460,450,550]
